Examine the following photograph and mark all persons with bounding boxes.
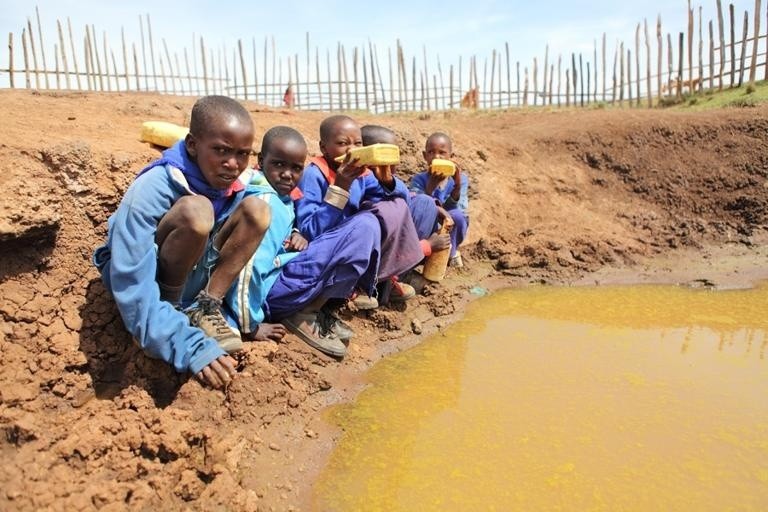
[94,94,271,387]
[225,126,381,358]
[296,114,425,311]
[358,126,456,305]
[409,132,470,276]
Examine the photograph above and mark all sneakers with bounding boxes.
[184,250,464,357]
